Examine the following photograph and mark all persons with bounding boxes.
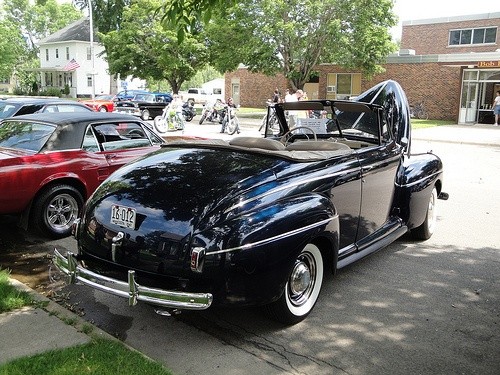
[272,88,308,134]
[492,91,500,126]
[191,101,194,106]
[220,97,240,134]
[168,95,185,129]
[212,99,225,121]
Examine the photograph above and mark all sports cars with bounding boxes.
[51,80,449,327]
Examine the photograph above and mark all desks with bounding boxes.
[479,109,495,124]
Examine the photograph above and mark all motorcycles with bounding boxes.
[153,96,241,137]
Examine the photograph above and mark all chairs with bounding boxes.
[285,141,350,151]
[229,137,286,152]
[83,134,97,147]
[153,97,162,102]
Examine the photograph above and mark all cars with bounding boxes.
[83,93,116,110]
[0,112,208,239]
[114,90,202,121]
[0,100,95,128]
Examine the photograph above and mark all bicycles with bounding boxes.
[258,99,287,132]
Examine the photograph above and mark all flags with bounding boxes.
[63,58,80,72]
